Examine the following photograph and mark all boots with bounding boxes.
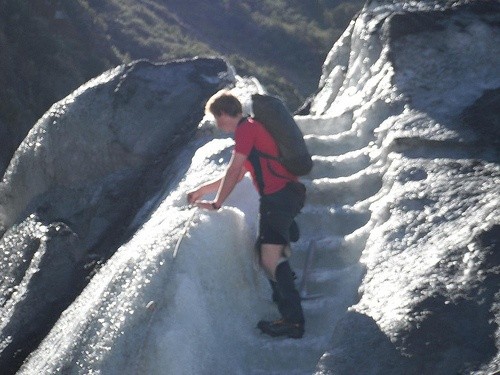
[256,258,304,339]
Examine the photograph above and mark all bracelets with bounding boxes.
[211,200,221,210]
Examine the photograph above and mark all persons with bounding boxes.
[186,93,306,338]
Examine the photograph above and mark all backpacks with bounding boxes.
[237,92,314,176]
